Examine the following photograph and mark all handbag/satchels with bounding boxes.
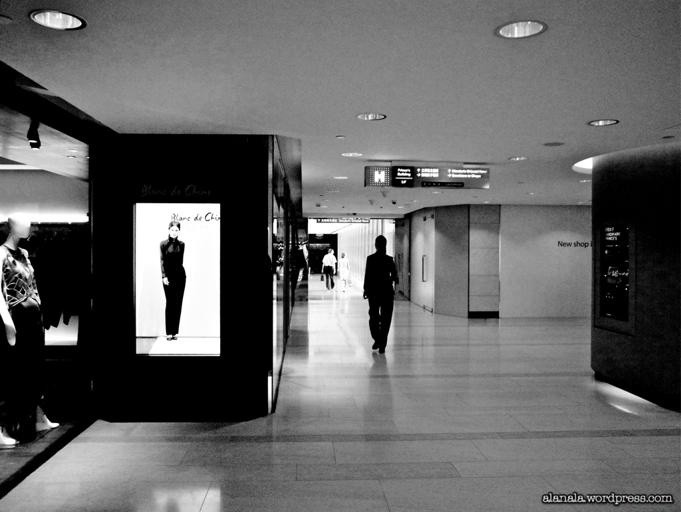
[321,275,324,281]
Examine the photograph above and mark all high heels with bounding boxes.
[166,336,178,341]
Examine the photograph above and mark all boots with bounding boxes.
[0,426,20,450]
[35,414,60,433]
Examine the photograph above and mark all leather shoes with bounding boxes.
[373,342,386,354]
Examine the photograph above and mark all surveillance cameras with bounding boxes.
[391,200,397,205]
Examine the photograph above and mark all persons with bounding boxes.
[363,235,399,353]
[0,212,60,446]
[160,221,186,341]
[321,248,350,292]
